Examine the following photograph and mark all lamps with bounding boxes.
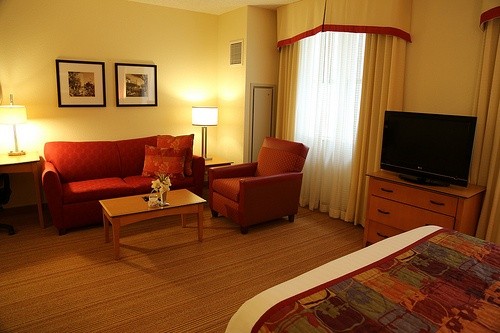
[191,106,218,160]
[0,94,27,156]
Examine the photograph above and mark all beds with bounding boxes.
[221,226,500,333]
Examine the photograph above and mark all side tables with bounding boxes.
[197,154,234,182]
[0,150,45,229]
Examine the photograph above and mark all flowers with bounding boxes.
[151,174,172,193]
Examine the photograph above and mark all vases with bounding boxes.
[160,192,167,202]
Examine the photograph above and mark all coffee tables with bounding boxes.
[100,190,207,261]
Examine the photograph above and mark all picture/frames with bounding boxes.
[56,59,106,107]
[114,62,158,106]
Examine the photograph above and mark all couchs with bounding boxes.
[209,137,309,235]
[41,136,206,237]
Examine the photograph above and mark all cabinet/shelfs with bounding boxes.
[363,171,486,247]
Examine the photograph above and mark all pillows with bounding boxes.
[142,145,186,179]
[157,134,194,175]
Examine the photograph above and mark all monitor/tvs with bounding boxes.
[380,110,477,186]
